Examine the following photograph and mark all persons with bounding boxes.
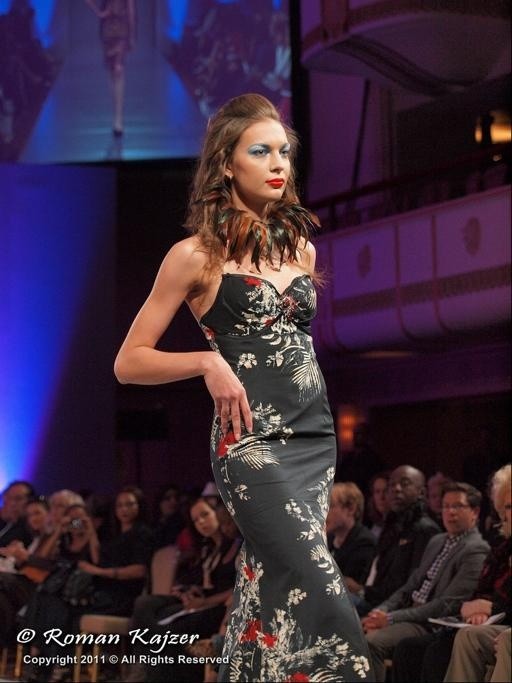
[0,463,512,681]
[88,1,139,139]
[0,0,63,161]
[111,93,375,683]
[157,0,291,127]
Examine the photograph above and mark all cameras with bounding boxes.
[68,518,82,528]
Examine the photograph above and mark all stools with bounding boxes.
[72,613,132,683]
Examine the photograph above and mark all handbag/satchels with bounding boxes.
[43,562,99,607]
